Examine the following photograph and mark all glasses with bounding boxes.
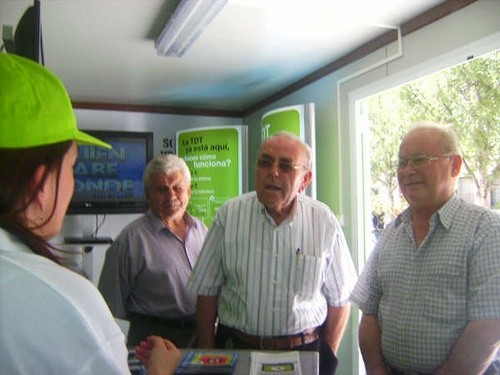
[256,160,305,173]
[398,153,453,169]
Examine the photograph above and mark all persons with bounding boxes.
[96,154,211,351]
[371,201,386,242]
[0,43,183,375]
[348,122,499,375]
[186,129,356,374]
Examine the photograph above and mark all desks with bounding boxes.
[129,350,320,375]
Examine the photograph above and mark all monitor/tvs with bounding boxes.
[12,0,44,66]
[66,127,155,214]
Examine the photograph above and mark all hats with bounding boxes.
[0,49,112,150]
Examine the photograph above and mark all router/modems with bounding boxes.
[64,235,112,244]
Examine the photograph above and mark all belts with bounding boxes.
[231,330,319,350]
[128,314,196,328]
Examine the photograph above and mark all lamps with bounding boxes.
[154,0,227,59]
